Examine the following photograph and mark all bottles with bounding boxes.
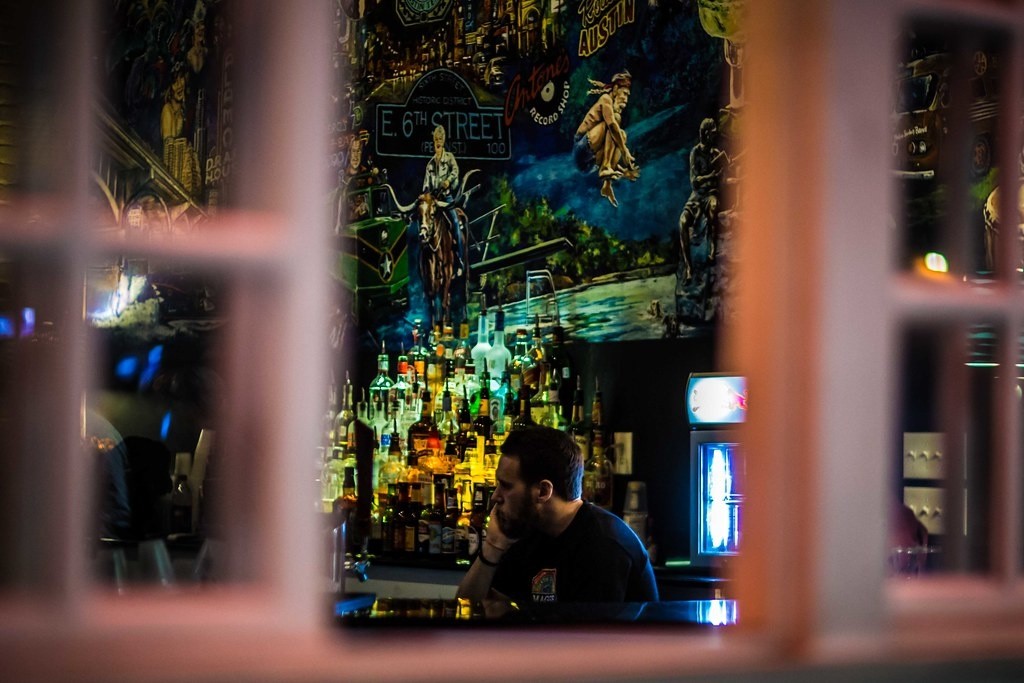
[325,286,653,574]
[169,447,204,536]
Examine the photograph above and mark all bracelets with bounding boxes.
[478,548,499,568]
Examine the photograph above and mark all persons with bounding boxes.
[676,116,724,282]
[186,1,209,71]
[422,125,465,276]
[449,424,663,616]
[572,70,640,208]
[335,137,378,237]
[161,61,189,142]
